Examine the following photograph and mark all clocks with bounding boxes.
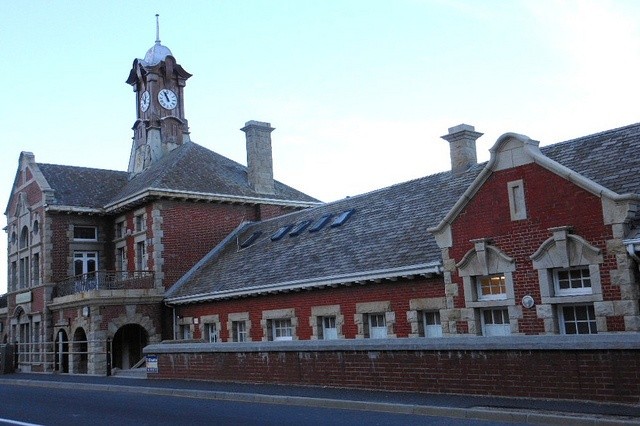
[157,88,178,111]
[139,90,151,113]
